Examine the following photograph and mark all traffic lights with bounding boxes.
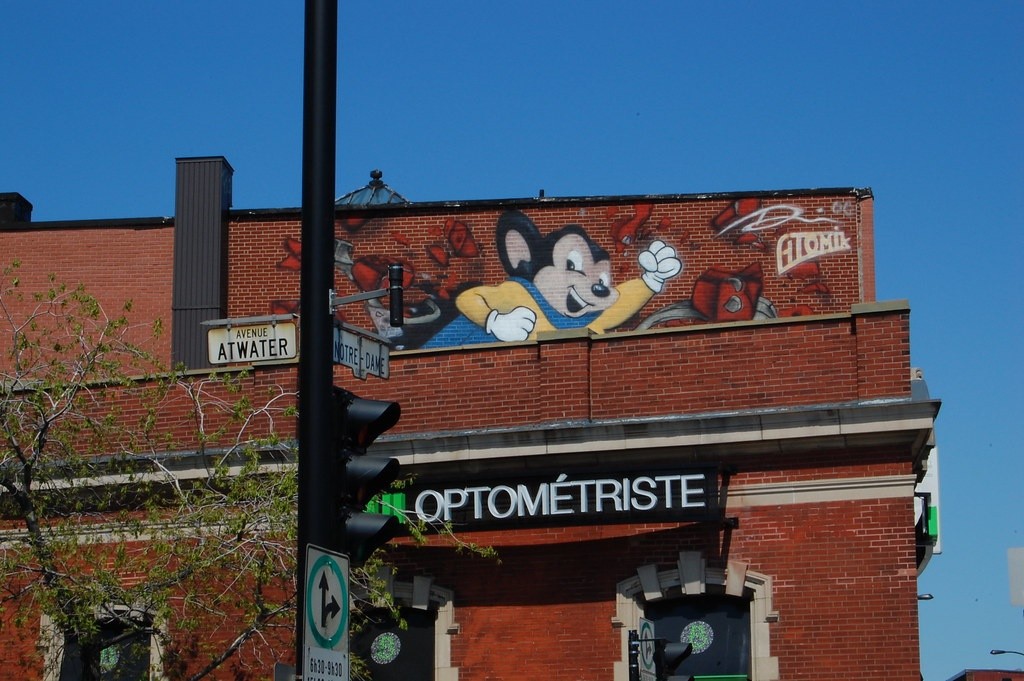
[654,635,694,679]
[332,387,404,566]
